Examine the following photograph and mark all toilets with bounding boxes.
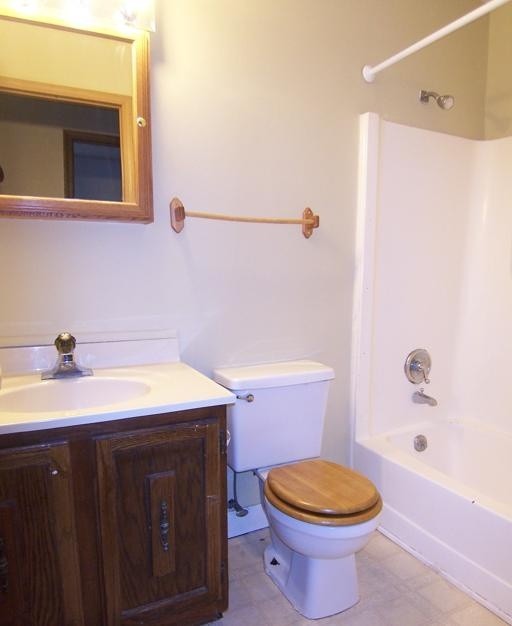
[213,360,384,621]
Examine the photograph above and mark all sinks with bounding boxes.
[0,371,151,414]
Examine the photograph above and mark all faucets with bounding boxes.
[40,331,94,380]
[413,387,438,407]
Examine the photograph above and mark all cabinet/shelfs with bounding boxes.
[0,434,91,625]
[91,403,227,625]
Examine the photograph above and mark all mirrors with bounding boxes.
[0,11,155,223]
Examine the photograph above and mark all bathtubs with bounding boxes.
[355,417,511,626]
